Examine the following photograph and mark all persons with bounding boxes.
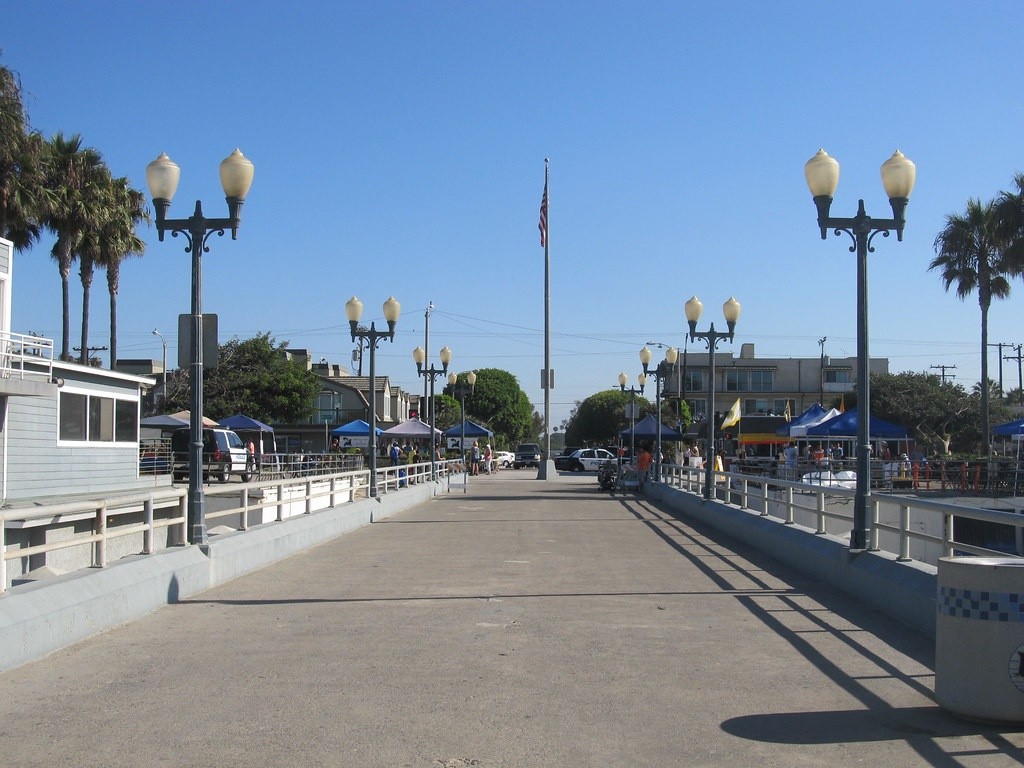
[469,441,479,476]
[247,438,254,456]
[987,451,999,489]
[381,438,446,487]
[483,444,492,475]
[653,441,746,475]
[771,441,843,476]
[618,446,624,466]
[636,447,652,491]
[330,439,339,453]
[870,441,955,477]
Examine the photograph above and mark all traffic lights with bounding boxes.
[409,409,419,420]
[726,434,732,440]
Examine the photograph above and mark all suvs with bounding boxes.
[515,443,545,469]
[480,451,515,468]
[170,428,255,483]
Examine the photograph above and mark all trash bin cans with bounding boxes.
[933,555,1024,725]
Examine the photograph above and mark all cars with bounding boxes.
[555,448,631,472]
[139,457,172,475]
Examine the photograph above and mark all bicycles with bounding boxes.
[281,448,327,479]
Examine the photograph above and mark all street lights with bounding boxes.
[684,295,741,498]
[345,294,401,497]
[152,329,167,399]
[640,345,678,481]
[448,371,477,463]
[619,371,646,464]
[413,345,452,480]
[804,148,918,547]
[817,336,828,408]
[148,146,256,545]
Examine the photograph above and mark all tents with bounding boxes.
[380,417,496,457]
[328,419,382,457]
[775,403,910,465]
[140,410,219,448]
[987,417,1024,497]
[214,414,280,472]
[618,414,682,474]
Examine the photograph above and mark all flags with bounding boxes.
[720,398,741,430]
[784,399,791,423]
